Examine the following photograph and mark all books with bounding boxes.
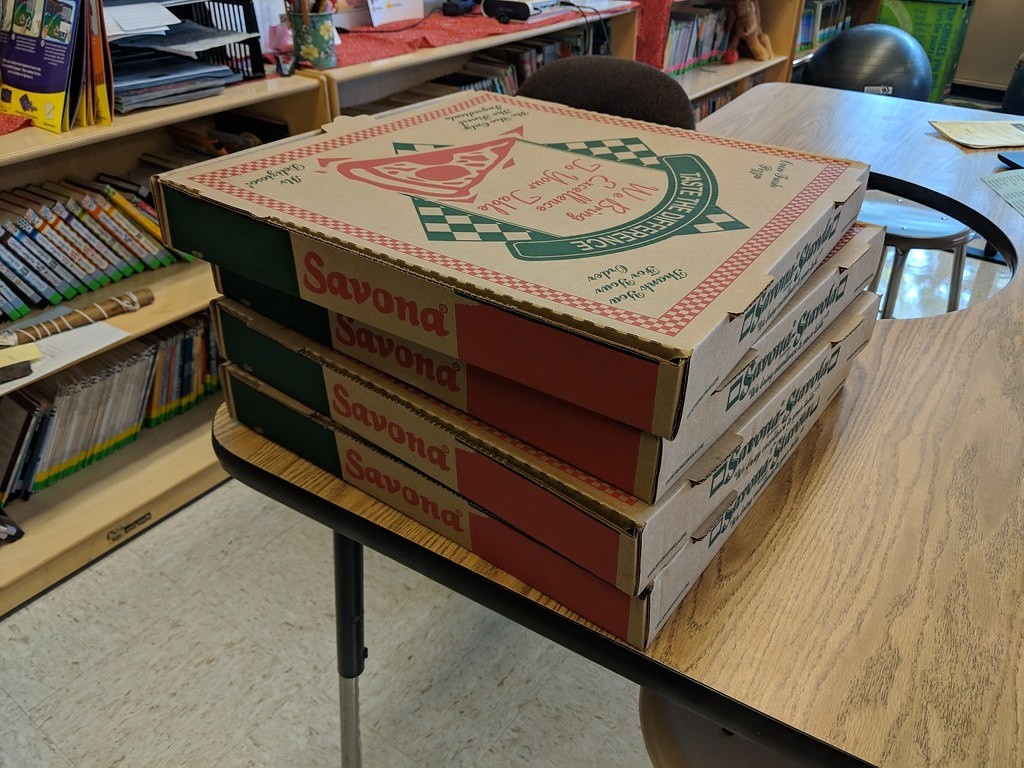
[663,6,734,73]
[340,25,594,116]
[692,71,769,126]
[0,309,220,510]
[0,113,289,320]
[796,0,851,52]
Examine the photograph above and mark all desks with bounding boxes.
[211,81,1023,767]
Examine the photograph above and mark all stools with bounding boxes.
[639,686,802,768]
[854,189,976,320]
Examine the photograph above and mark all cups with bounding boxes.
[289,12,337,71]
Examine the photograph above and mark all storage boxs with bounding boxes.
[875,0,975,104]
[147,90,886,654]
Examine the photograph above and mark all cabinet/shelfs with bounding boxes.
[0,65,329,620]
[307,0,639,122]
[634,0,852,122]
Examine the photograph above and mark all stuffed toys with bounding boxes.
[725,0,774,61]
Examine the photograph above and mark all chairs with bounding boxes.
[516,55,695,130]
